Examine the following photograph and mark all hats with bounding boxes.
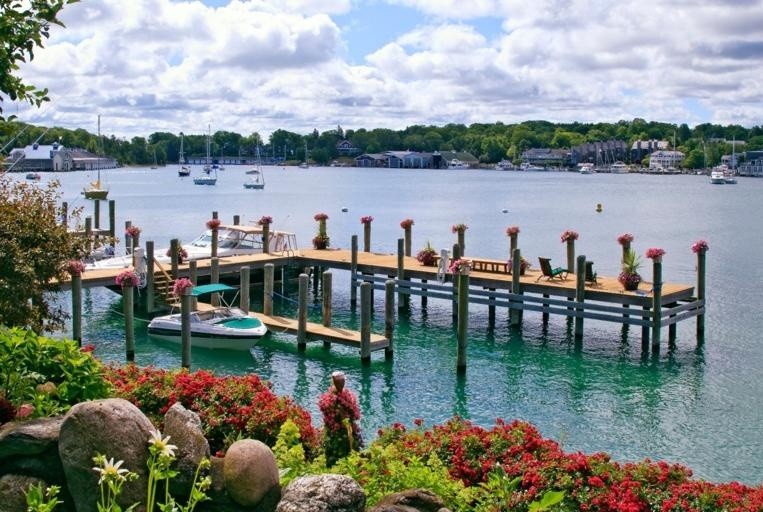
[332,372,346,380]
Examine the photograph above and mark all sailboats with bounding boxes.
[577,130,737,184]
[171,123,309,190]
[80,115,110,200]
[150,148,158,169]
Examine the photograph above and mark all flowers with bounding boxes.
[451,256,472,274]
[313,232,330,247]
[616,232,634,244]
[618,273,642,284]
[360,215,374,225]
[126,224,142,237]
[65,261,87,275]
[166,241,189,258]
[172,278,193,295]
[205,218,221,231]
[314,213,329,222]
[115,269,141,290]
[691,239,708,253]
[506,224,520,236]
[560,228,578,242]
[644,247,666,260]
[257,214,273,226]
[451,222,469,234]
[507,253,532,270]
[400,219,414,229]
[417,240,439,262]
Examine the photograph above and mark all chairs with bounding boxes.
[536,256,571,283]
[586,262,598,288]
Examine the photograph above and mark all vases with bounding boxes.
[623,282,638,291]
[519,268,526,275]
[424,258,434,266]
[177,258,184,264]
[317,244,326,250]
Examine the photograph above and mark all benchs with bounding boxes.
[472,258,511,273]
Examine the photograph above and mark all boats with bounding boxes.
[495,159,513,170]
[77,223,319,300]
[446,158,470,170]
[146,282,268,352]
[519,162,534,171]
[25,173,41,180]
